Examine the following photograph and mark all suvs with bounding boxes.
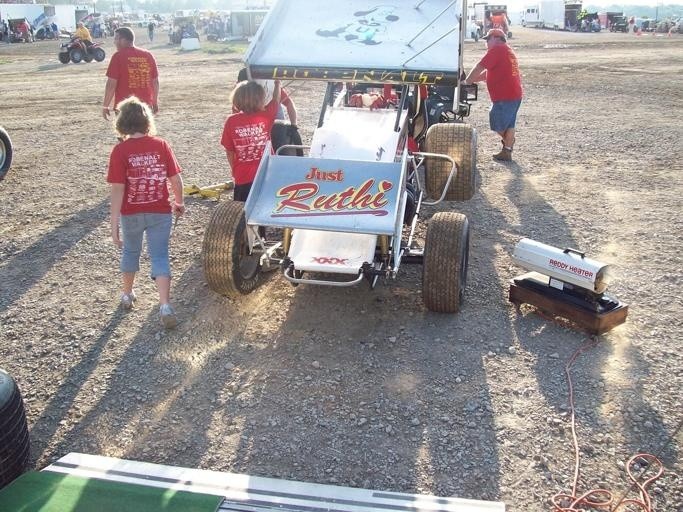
[203,0,480,317]
[36,26,60,39]
[58,36,105,64]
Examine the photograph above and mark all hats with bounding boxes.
[483,29,505,39]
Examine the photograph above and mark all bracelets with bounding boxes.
[175,201,184,207]
[103,107,109,109]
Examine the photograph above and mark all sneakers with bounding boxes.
[121,290,137,310]
[502,137,515,152]
[159,304,177,329]
[493,149,512,161]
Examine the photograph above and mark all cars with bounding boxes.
[609,15,628,32]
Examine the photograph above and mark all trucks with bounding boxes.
[170,15,199,44]
[6,16,36,44]
[473,3,511,38]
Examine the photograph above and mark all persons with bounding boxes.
[50,22,59,40]
[629,16,635,32]
[102,28,159,122]
[220,79,281,201]
[460,28,523,162]
[147,22,154,41]
[71,21,93,48]
[232,68,296,126]
[107,96,186,329]
